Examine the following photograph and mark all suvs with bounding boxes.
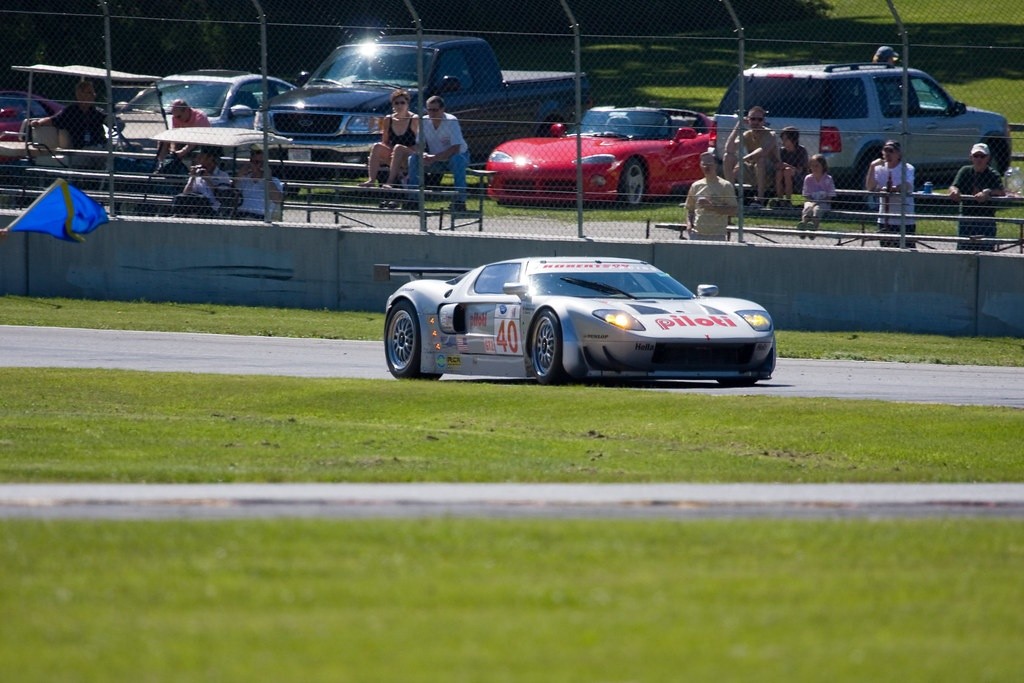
[712,47,1013,204]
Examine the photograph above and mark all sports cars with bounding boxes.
[485,106,715,204]
[375,257,777,388]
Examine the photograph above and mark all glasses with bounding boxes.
[748,116,765,121]
[249,150,262,156]
[884,149,896,153]
[425,106,441,113]
[394,101,405,105]
[973,154,988,159]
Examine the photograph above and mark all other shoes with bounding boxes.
[749,197,766,207]
[772,199,793,210]
[402,199,418,210]
[447,201,466,211]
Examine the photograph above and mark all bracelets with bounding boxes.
[989,189,992,196]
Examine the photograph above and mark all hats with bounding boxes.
[970,143,990,156]
[883,139,901,150]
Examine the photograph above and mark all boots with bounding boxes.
[797,215,820,240]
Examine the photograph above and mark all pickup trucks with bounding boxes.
[253,35,589,179]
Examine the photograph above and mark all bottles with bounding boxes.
[923,182,933,195]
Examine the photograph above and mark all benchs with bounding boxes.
[0,139,498,233]
[653,171,1024,257]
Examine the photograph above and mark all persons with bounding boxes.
[357,89,419,188]
[684,152,737,241]
[236,150,283,221]
[184,145,230,211]
[948,143,1006,252]
[772,127,808,207]
[722,106,781,206]
[865,141,916,248]
[872,46,899,62]
[169,101,229,172]
[796,154,835,240]
[30,81,128,191]
[403,96,470,211]
[605,116,634,135]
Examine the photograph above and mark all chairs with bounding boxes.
[605,115,636,133]
[658,117,689,135]
[61,130,74,167]
[231,90,260,109]
[30,124,59,167]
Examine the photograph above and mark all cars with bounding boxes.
[106,67,298,169]
[0,90,71,164]
